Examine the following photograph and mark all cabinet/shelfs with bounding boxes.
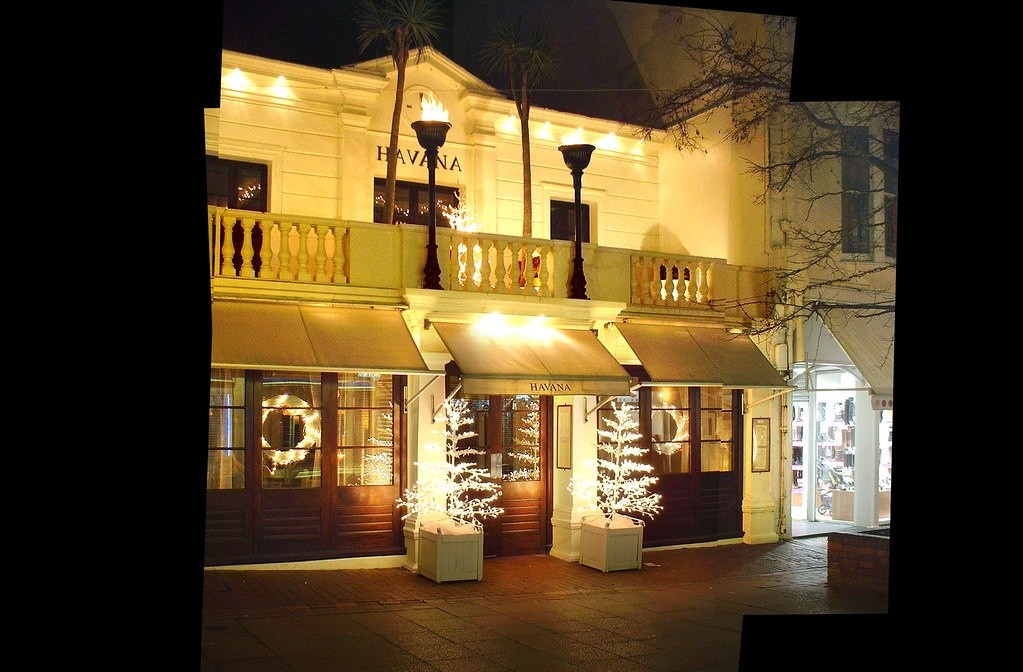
[793,419,842,492]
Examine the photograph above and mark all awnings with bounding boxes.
[422,318,633,424]
[582,320,798,425]
[795,300,893,411]
[209,298,447,412]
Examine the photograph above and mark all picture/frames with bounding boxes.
[556,404,572,470]
[708,411,716,444]
[752,418,771,471]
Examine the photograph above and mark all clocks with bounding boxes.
[402,86,441,125]
